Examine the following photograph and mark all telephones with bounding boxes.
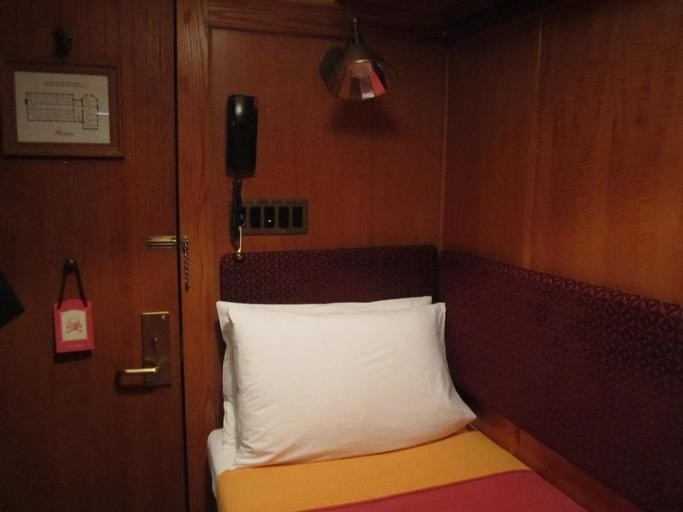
[227,95,257,171]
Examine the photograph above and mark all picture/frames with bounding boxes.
[0,59,123,159]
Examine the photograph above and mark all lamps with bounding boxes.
[318,17,399,101]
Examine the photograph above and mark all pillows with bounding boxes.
[225,300,479,467]
[214,293,435,457]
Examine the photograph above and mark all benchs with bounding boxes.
[205,244,682,512]
